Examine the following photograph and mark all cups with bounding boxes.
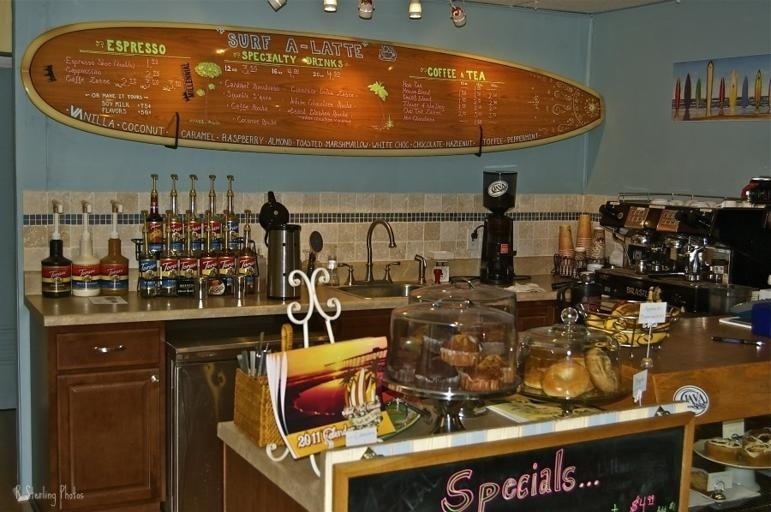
[557,215,606,277]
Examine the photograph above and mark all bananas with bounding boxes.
[584,287,684,347]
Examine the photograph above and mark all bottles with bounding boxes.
[140,209,256,299]
[204,188,222,253]
[183,189,203,257]
[325,255,341,287]
[218,190,240,253]
[98,201,130,298]
[145,190,165,257]
[70,199,108,297]
[39,198,74,298]
[166,189,185,257]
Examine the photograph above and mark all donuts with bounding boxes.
[523,347,617,397]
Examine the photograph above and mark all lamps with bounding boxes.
[265,1,469,29]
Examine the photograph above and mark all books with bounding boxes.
[266,334,426,462]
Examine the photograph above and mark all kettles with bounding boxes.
[255,190,303,303]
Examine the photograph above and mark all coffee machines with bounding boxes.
[598,194,770,288]
[467,167,520,285]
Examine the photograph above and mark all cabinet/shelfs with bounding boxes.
[51,323,169,511]
[514,299,569,336]
[321,311,391,341]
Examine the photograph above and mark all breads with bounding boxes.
[704,426,771,467]
[387,333,514,399]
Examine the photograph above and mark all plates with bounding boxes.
[690,438,771,472]
[689,465,762,510]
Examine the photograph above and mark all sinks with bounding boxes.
[328,282,423,300]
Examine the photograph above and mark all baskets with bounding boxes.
[576,300,685,357]
[554,254,611,283]
[234,367,286,448]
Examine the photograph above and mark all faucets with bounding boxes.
[366,219,396,283]
[415,253,427,283]
